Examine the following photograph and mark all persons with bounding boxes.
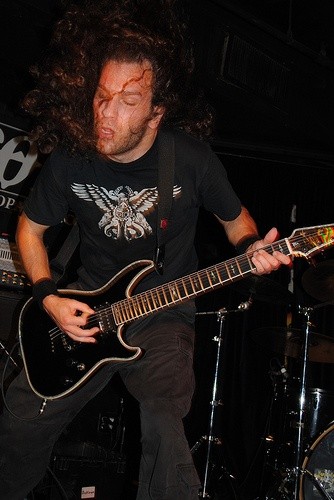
[0,0,292,500]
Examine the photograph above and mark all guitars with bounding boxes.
[19,224,334,400]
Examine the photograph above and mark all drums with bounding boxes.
[299,419,334,500]
[289,387,307,429]
[303,388,334,438]
[273,377,303,400]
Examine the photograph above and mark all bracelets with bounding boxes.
[32,279,56,310]
[237,234,261,254]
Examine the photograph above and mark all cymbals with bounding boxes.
[250,328,334,363]
[230,273,295,306]
[302,259,334,305]
